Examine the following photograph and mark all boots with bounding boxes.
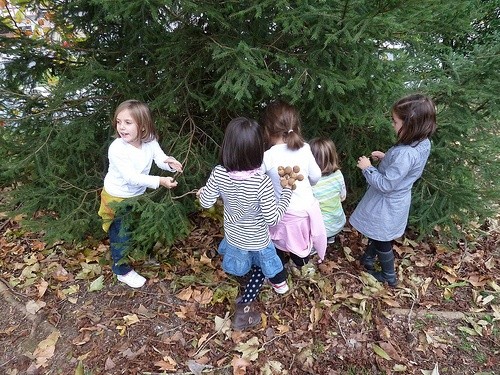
[231,302,261,331]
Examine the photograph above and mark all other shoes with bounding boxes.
[266,280,290,294]
[356,254,375,268]
[372,271,398,286]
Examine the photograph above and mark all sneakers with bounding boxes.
[116,270,146,289]
